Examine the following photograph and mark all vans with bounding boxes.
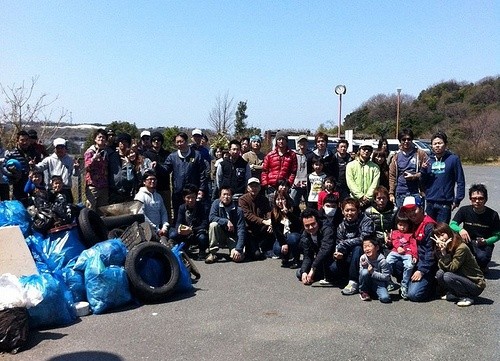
[271,135,431,167]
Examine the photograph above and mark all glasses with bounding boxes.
[141,137,149,141]
[401,138,411,141]
[152,138,160,142]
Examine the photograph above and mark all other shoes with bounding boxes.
[205,253,218,264]
[440,293,459,300]
[311,279,333,287]
[456,297,474,306]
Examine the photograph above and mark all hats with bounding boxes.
[275,130,289,140]
[192,129,203,137]
[140,130,151,138]
[359,141,373,152]
[150,132,165,145]
[296,135,309,143]
[52,137,67,148]
[399,195,424,213]
[249,135,261,144]
[246,177,261,185]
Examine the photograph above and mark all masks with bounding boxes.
[323,205,336,217]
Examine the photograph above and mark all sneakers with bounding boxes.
[359,290,371,301]
[399,287,408,300]
[387,281,395,292]
[340,279,359,295]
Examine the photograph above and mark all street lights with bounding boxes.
[396,88,402,139]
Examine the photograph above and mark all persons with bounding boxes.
[0,128,439,303]
[429,221,486,306]
[420,131,465,225]
[449,183,500,272]
